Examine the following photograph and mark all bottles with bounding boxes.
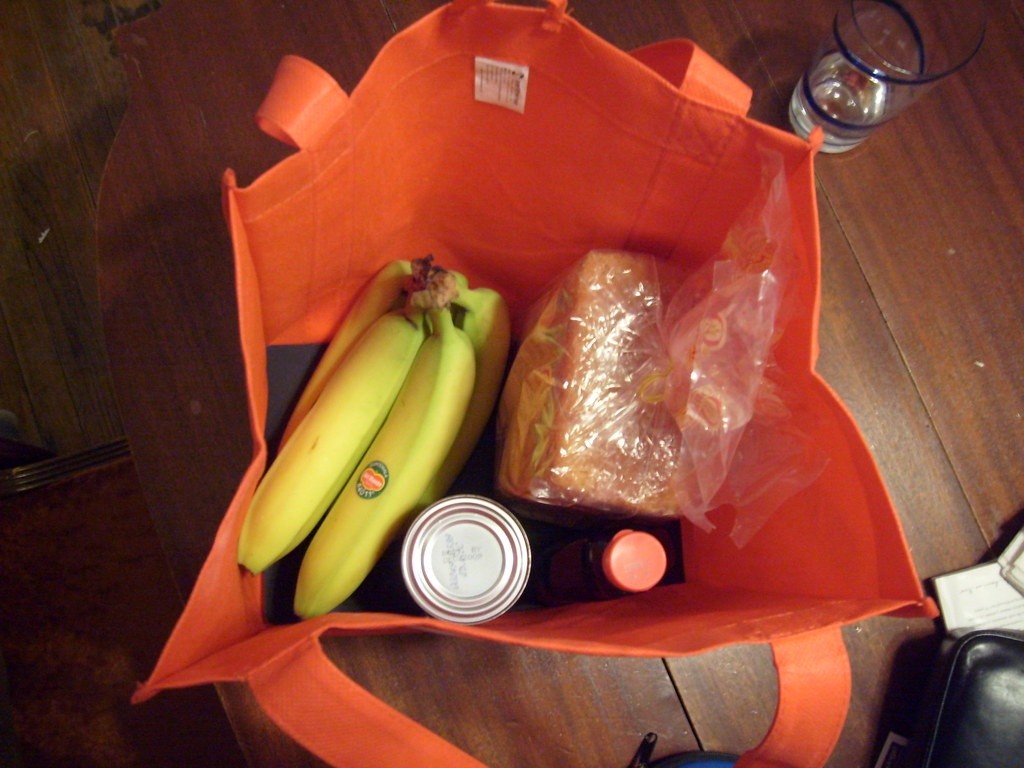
[527,530,667,603]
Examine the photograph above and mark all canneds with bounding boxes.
[400,494,532,626]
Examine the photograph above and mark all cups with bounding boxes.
[788,0,985,152]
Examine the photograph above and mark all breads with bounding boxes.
[492,249,709,519]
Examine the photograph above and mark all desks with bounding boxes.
[96,1,1024,768]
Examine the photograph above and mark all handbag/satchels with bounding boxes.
[130,0,941,768]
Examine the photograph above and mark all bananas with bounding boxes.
[237,255,510,617]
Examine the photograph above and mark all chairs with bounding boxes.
[0,438,246,768]
